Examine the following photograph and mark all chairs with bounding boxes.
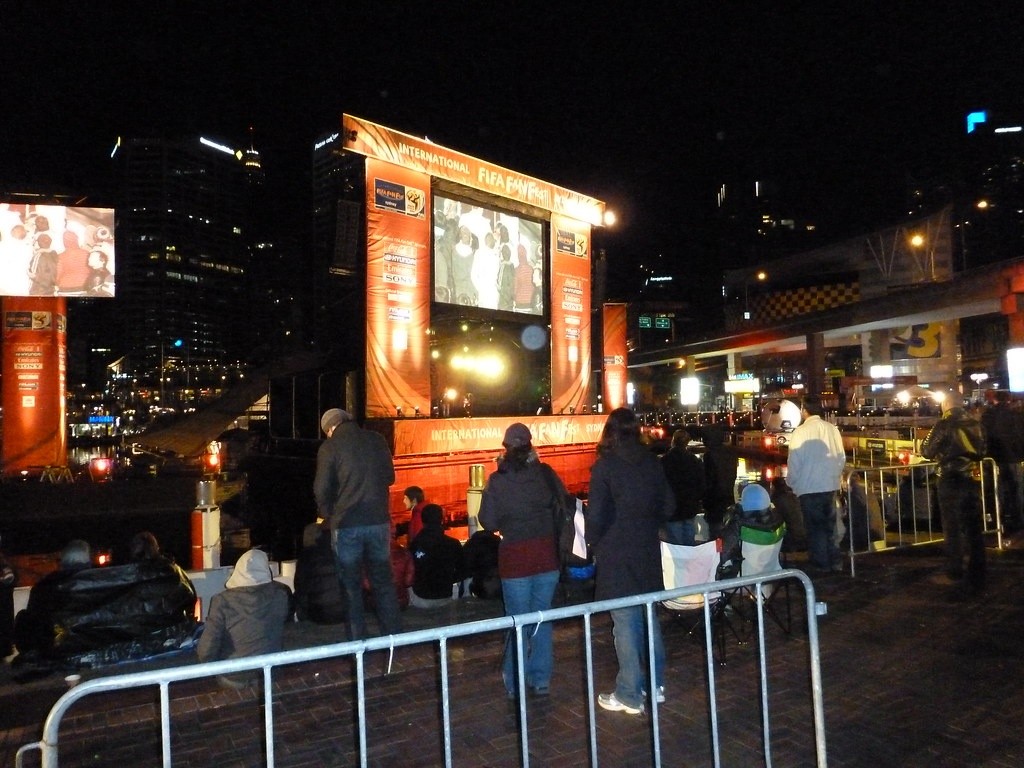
[659,522,793,666]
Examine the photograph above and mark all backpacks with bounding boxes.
[937,415,988,482]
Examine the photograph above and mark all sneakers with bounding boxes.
[641,685,667,703]
[598,693,644,714]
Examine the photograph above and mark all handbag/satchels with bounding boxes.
[540,463,576,562]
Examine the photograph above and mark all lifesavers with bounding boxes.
[777,436,787,444]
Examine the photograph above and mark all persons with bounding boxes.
[0,486,592,686]
[588,407,678,714]
[314,408,406,657]
[478,424,570,698]
[0,203,115,298]
[650,387,1024,619]
[434,196,543,313]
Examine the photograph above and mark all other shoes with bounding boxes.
[831,562,845,573]
[929,574,962,585]
[961,554,970,571]
[506,687,520,701]
[526,681,550,697]
[802,560,830,573]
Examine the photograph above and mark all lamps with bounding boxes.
[396,405,439,418]
[560,405,597,415]
[90,457,114,484]
[347,131,357,141]
[202,441,221,480]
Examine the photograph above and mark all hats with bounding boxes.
[321,408,349,434]
[504,423,532,447]
[422,504,443,523]
[741,484,771,511]
